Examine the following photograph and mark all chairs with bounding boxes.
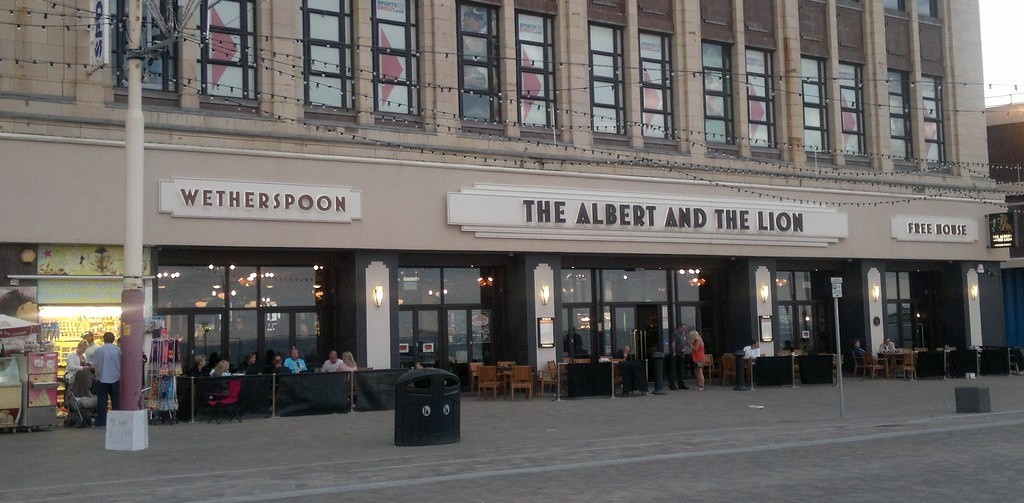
[703,347,958,385]
[467,357,625,401]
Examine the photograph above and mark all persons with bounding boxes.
[319,350,358,372]
[206,360,231,377]
[879,337,895,355]
[613,346,631,360]
[187,354,208,377]
[284,348,307,373]
[65,331,121,427]
[688,330,705,391]
[40,319,60,342]
[742,339,761,368]
[667,323,690,390]
[851,338,880,377]
[270,355,292,373]
[238,351,260,374]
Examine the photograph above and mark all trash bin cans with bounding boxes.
[394,361,461,446]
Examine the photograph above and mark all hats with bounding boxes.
[80,331,94,338]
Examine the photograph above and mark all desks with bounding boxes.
[495,366,512,399]
[878,351,918,378]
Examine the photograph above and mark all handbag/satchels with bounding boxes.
[696,361,703,368]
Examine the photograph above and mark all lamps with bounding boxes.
[971,285,977,301]
[872,286,879,301]
[374,285,384,307]
[540,286,549,305]
[761,285,768,302]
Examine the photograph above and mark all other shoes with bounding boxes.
[679,385,689,389]
[694,386,704,391]
